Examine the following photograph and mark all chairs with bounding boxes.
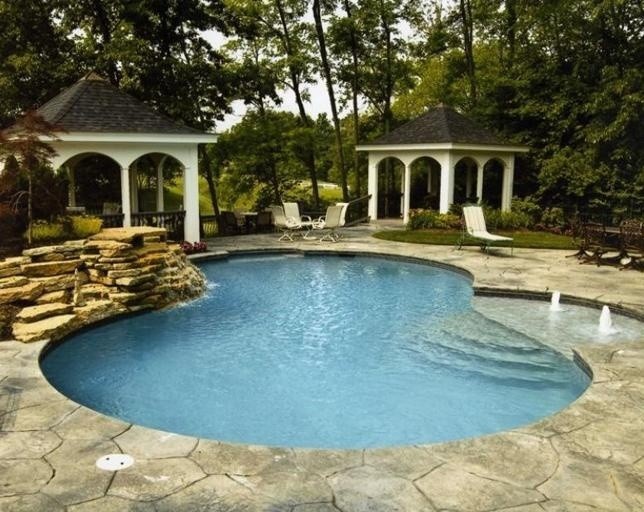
[565,217,644,272]
[221,211,271,236]
[458,206,513,256]
[268,202,350,243]
